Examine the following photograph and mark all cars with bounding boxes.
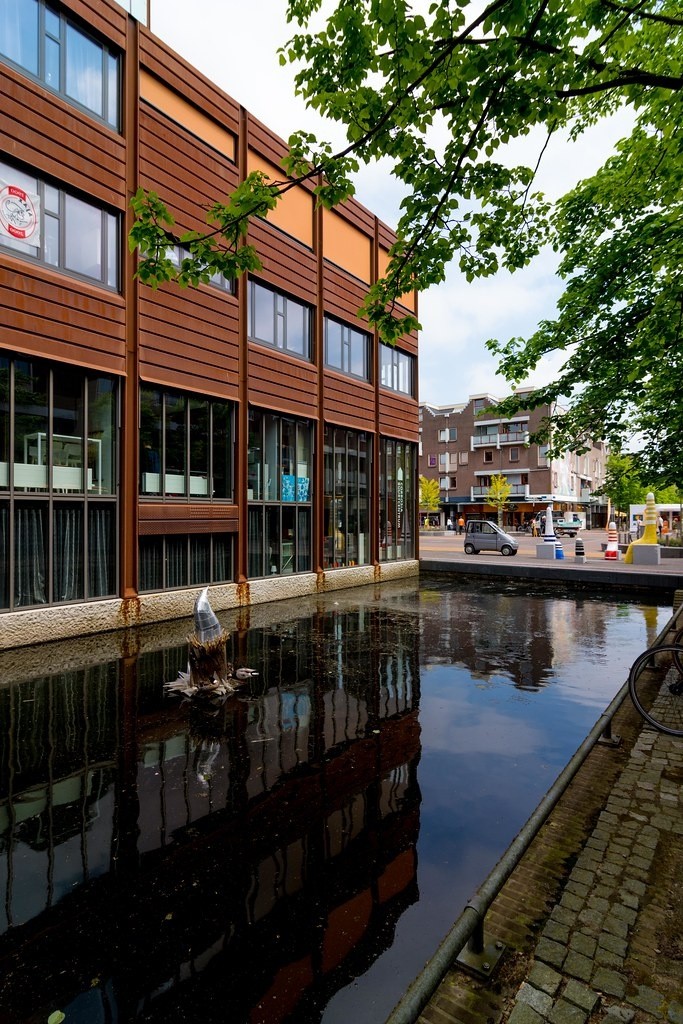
[464,520,519,556]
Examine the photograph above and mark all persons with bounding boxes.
[447,515,464,535]
[635,515,642,539]
[423,517,426,526]
[433,516,439,527]
[658,514,679,538]
[508,514,541,538]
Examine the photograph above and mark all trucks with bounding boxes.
[530,510,582,537]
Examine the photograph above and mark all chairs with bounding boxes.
[476,524,479,532]
[34,441,82,495]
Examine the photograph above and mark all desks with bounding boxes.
[23,430,103,495]
